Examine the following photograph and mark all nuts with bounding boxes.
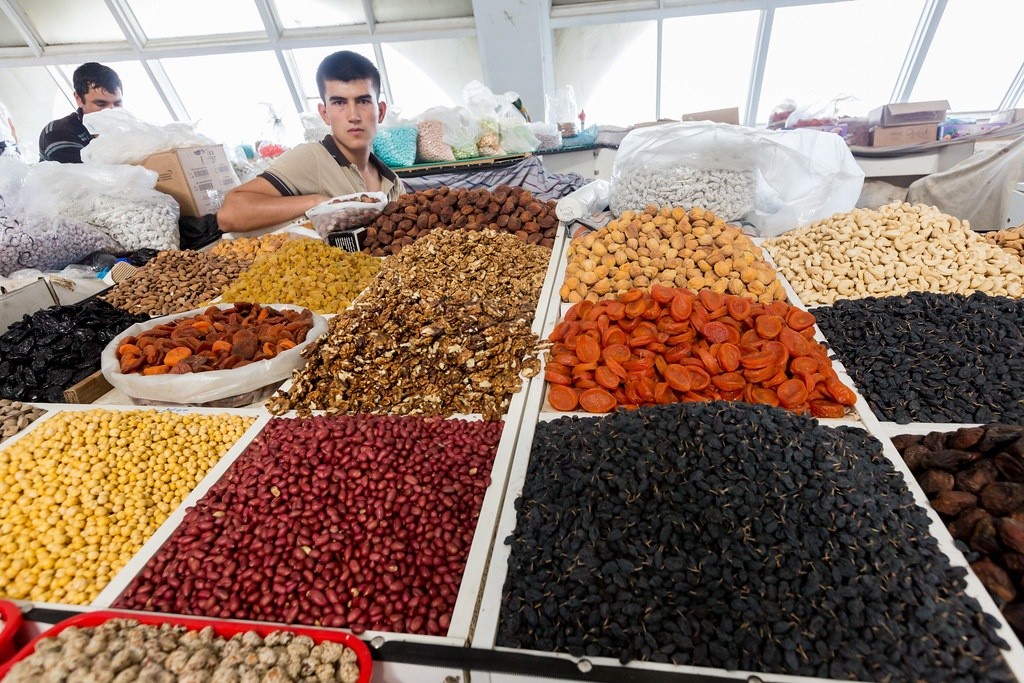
[560,199,1024,306]
[95,247,253,317]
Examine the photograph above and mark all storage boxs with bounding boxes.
[938,109,1016,141]
[777,124,846,139]
[0,218,1018,683]
[682,108,740,129]
[869,122,937,147]
[868,99,951,126]
[137,143,244,217]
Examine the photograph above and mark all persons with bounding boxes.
[38,63,123,163]
[0,102,21,157]
[217,51,406,233]
